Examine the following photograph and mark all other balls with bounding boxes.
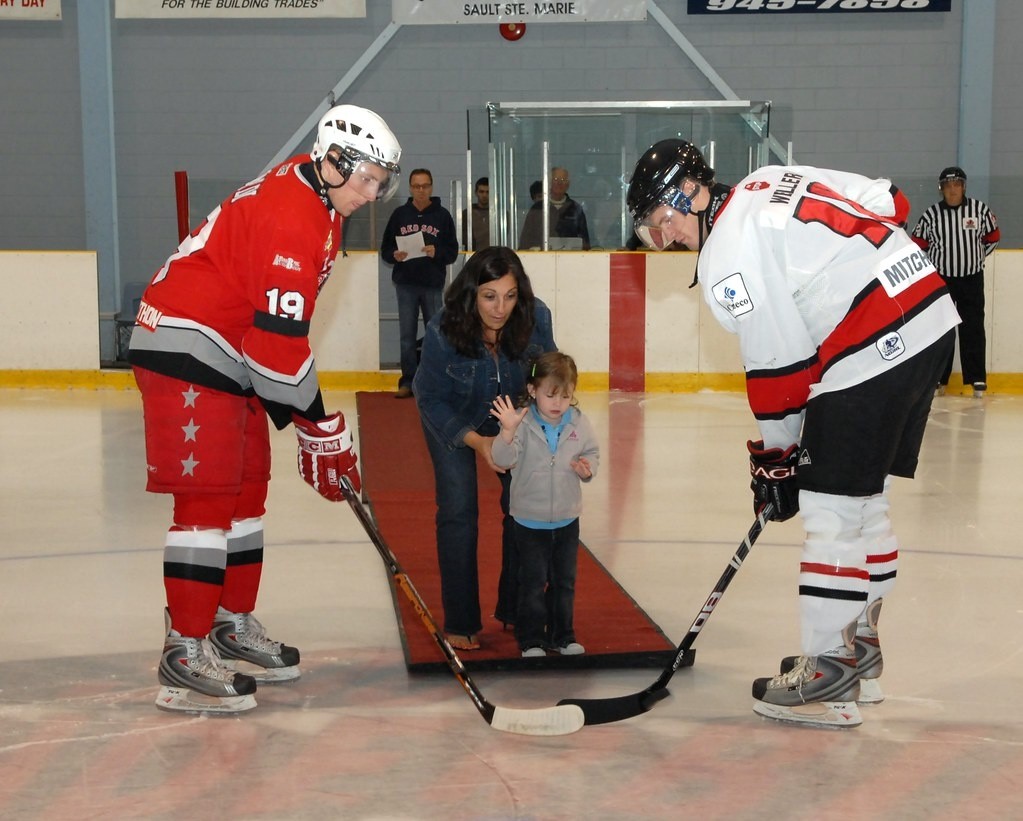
[641,687,671,708]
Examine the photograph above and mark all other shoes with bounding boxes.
[395,385,413,397]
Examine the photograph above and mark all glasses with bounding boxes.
[553,177,566,182]
[410,183,431,189]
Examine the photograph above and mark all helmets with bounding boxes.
[310,104,402,178]
[939,167,966,192]
[625,138,716,230]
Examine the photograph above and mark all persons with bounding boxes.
[490,352,599,658]
[625,139,964,727]
[626,236,688,250]
[531,181,542,202]
[127,103,401,714]
[912,167,1001,396]
[518,168,590,250]
[412,247,559,650]
[462,177,510,251]
[380,169,458,399]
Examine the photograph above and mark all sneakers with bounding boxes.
[972,381,987,397]
[522,643,546,657]
[155,606,258,715]
[548,640,585,656]
[933,382,946,396]
[207,606,301,686]
[780,597,885,705]
[751,622,863,731]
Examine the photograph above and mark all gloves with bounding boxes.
[291,412,360,502]
[747,440,801,522]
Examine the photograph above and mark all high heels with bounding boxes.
[447,632,480,651]
[502,621,551,634]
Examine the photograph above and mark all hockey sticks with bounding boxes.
[337,473,586,736]
[555,501,775,726]
